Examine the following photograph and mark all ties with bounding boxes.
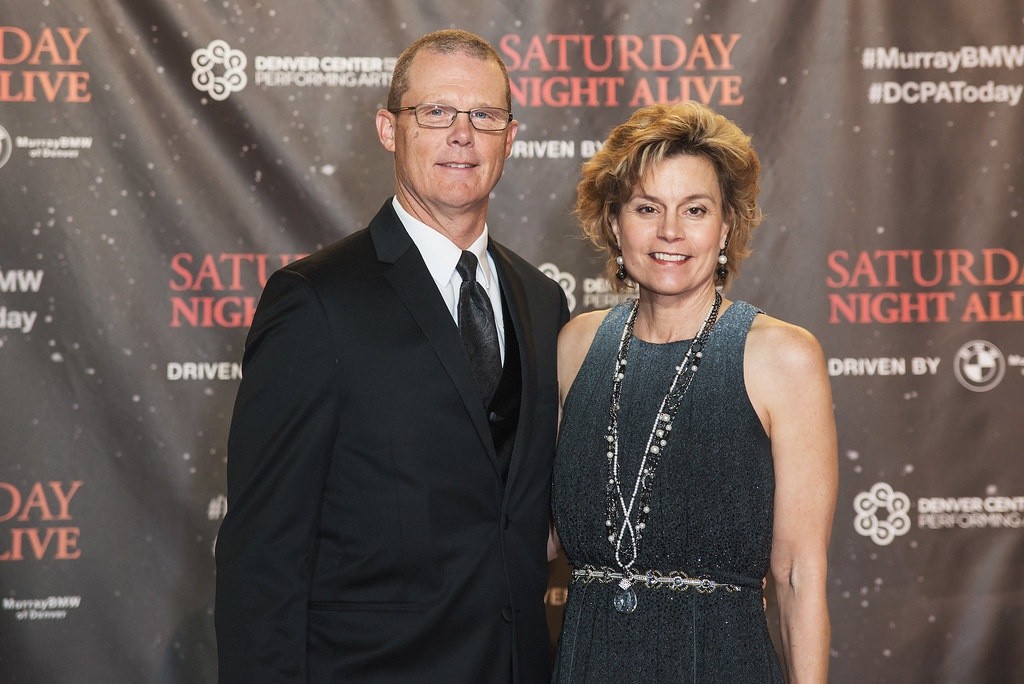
[456,250,502,414]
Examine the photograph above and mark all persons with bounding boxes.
[550,98,839,684]
[215,28,769,684]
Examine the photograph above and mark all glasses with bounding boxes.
[388,102,514,132]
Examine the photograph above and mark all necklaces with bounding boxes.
[604,289,722,613]
